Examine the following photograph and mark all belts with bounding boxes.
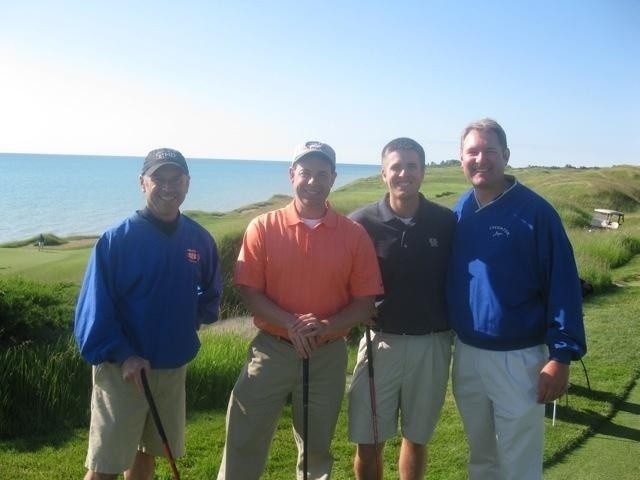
[274,334,295,349]
[366,324,453,337]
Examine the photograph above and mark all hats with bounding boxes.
[290,141,336,172]
[140,148,190,177]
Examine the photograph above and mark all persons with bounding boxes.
[451,118,587,479]
[74,148,224,480]
[345,137,459,479]
[219,140,385,479]
